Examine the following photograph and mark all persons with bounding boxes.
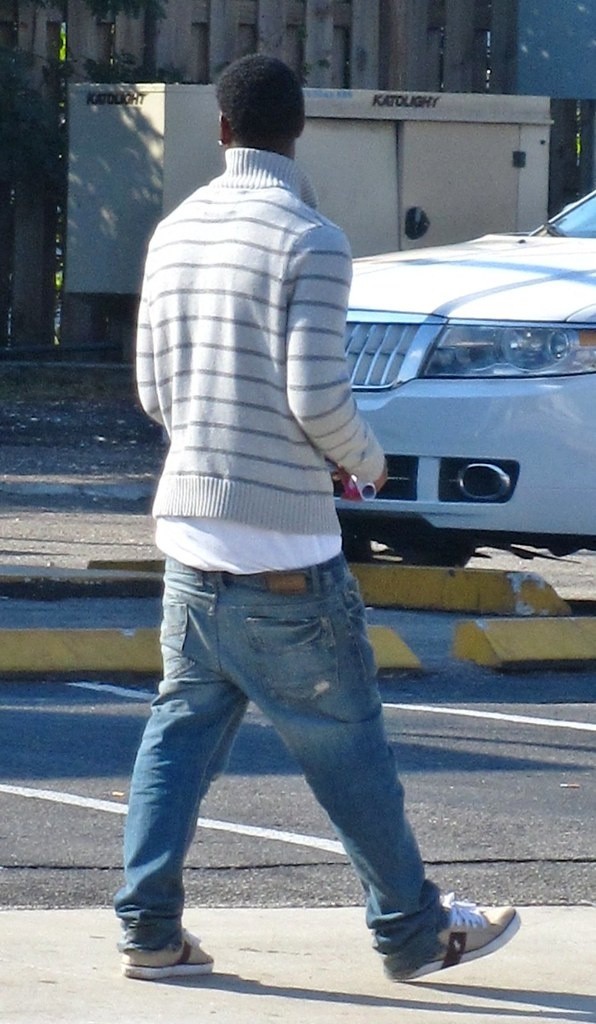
[111,55,521,985]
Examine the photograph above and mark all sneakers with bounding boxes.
[380,893,522,983]
[124,928,214,980]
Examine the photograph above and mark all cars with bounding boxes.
[325,181,596,567]
